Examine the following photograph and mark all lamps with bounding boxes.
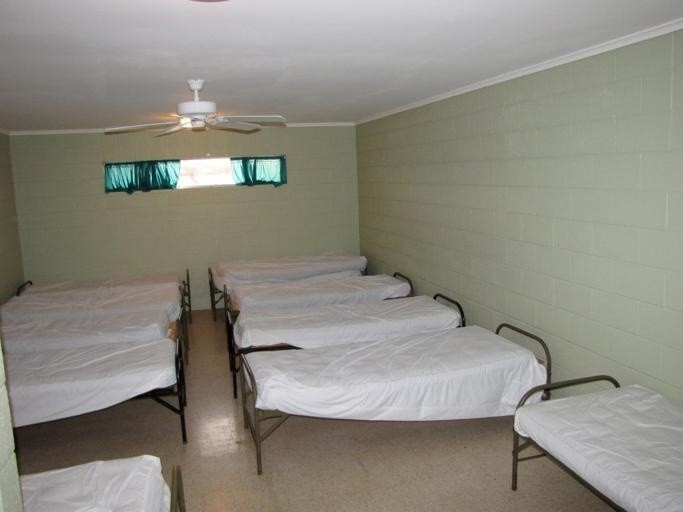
[169,112,216,129]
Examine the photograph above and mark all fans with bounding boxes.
[98,78,297,139]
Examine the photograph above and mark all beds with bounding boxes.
[2,268,195,443]
[20,454,190,512]
[509,373,681,512]
